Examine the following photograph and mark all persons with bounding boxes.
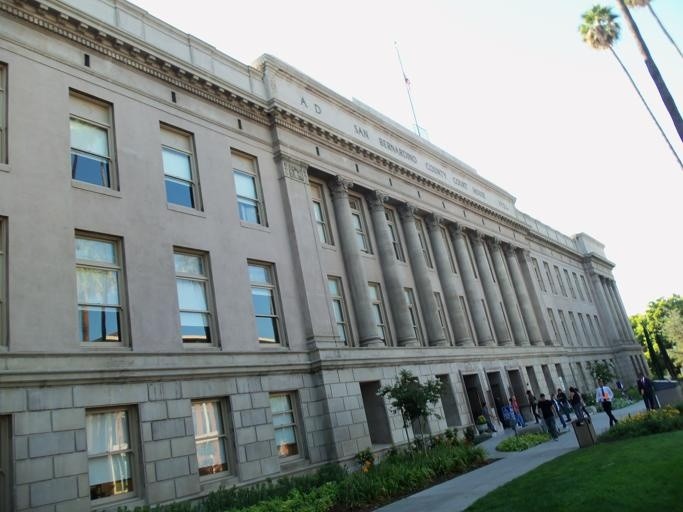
[595,379,618,429]
[551,394,566,429]
[575,388,591,420]
[637,373,655,412]
[509,396,528,428]
[537,393,559,441]
[557,388,572,422]
[526,390,541,424]
[496,398,509,428]
[567,387,583,420]
[482,402,498,432]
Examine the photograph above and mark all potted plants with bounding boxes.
[475,413,490,434]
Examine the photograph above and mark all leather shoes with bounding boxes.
[519,418,573,441]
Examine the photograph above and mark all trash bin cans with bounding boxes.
[571,417,597,448]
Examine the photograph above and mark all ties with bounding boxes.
[601,388,606,401]
